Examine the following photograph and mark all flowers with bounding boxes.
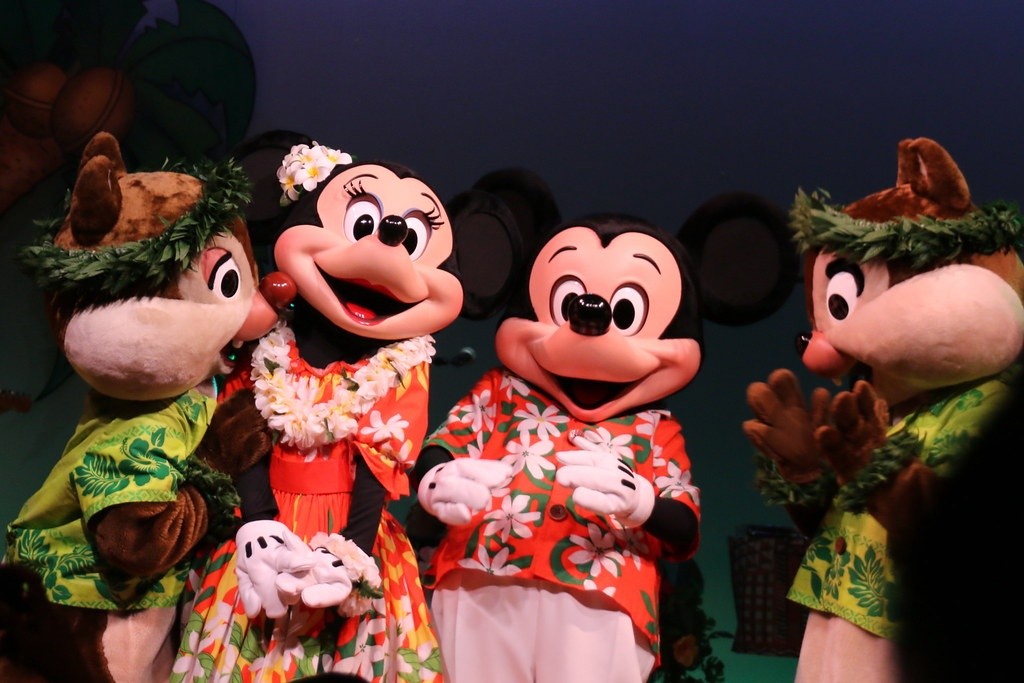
[275,139,352,205]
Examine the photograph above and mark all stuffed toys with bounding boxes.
[412,167,798,682]
[742,138,1024,683]
[0,130,298,683]
[167,129,518,682]
